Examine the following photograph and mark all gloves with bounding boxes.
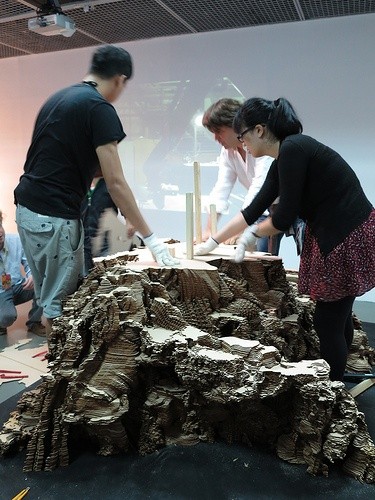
[194,238,219,257]
[235,225,258,262]
[143,232,180,268]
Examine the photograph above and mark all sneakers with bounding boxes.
[0,327,7,336]
[26,321,46,337]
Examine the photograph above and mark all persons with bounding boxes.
[14,45,180,350]
[183,97,375,380]
[0,211,46,337]
[193,97,305,252]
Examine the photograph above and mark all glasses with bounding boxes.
[237,124,266,143]
